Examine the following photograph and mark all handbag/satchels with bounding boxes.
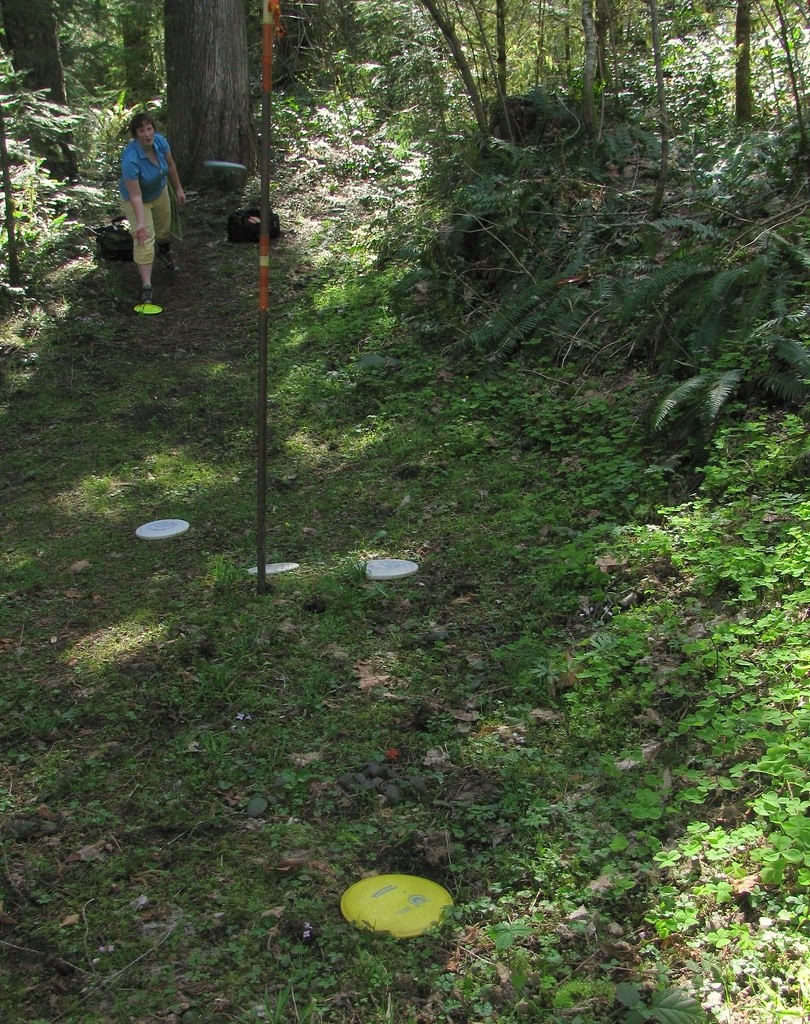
[97,228,134,261]
[228,207,280,243]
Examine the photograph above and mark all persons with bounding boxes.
[119,113,186,304]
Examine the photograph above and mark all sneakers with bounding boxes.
[141,285,154,304]
[155,248,180,272]
